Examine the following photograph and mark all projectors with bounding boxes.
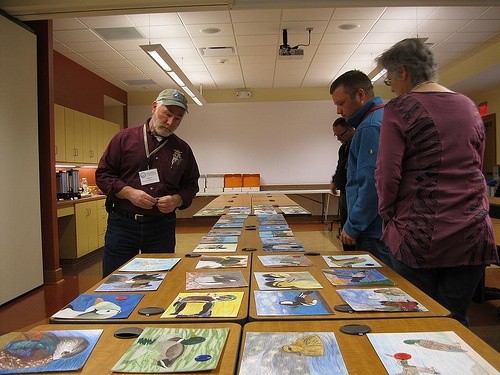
[278,48,304,61]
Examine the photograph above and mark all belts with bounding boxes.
[112,207,166,223]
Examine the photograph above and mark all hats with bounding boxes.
[155,88,188,114]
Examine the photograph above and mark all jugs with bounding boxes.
[57,169,81,200]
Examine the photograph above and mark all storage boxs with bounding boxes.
[205,173,224,193]
[197,174,206,193]
[224,173,242,192]
[242,174,260,192]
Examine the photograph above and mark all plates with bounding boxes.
[81,194,92,197]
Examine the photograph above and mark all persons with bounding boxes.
[375,36,499,326]
[330,67,387,266]
[95,89,199,279]
[331,117,354,228]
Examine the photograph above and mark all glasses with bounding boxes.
[334,127,349,139]
[383,73,394,86]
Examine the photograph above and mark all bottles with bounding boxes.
[81,178,88,194]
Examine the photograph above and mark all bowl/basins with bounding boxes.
[88,186,99,192]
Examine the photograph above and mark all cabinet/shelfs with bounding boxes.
[58,199,108,264]
[54,104,120,163]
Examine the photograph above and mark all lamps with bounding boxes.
[139,12,207,108]
[367,6,436,84]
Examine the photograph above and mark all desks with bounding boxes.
[1,251,500,375]
[196,187,342,227]
[192,214,305,252]
[194,192,314,215]
